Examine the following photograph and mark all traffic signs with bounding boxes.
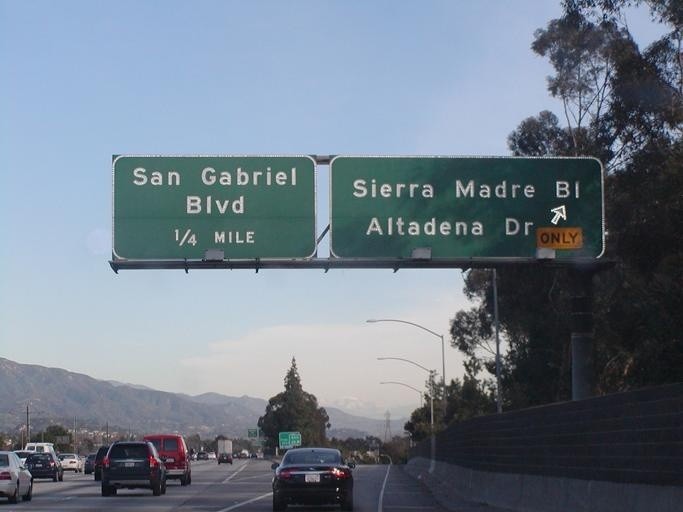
[325,152,608,268]
[278,432,294,451]
[109,149,318,268]
[247,428,257,437]
[288,434,302,447]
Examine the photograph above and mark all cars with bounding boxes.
[268,446,357,512]
[0,434,265,505]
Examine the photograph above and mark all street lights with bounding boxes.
[364,317,451,465]
